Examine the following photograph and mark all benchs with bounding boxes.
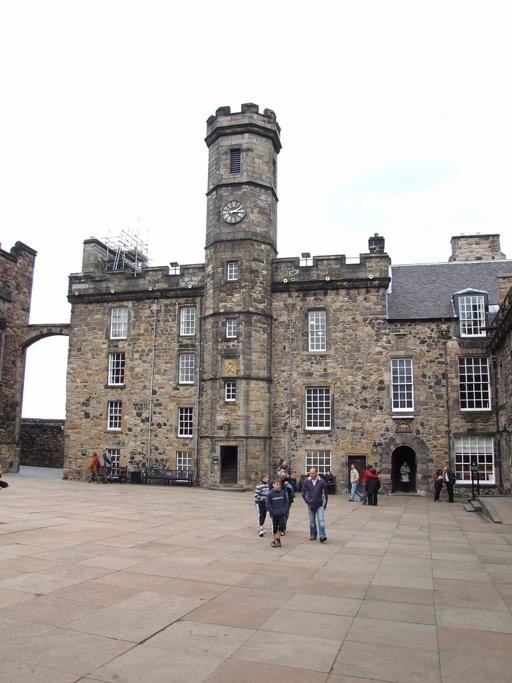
[90,464,128,483]
[141,467,194,487]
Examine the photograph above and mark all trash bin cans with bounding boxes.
[130,471,142,484]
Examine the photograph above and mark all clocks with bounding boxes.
[221,199,248,224]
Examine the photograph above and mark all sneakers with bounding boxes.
[320,537,328,543]
[258,528,266,536]
[433,498,454,503]
[348,495,378,506]
[271,540,276,548]
[310,535,317,541]
[276,538,282,547]
[282,531,288,536]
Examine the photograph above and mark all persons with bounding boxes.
[365,470,380,506]
[301,468,328,542]
[254,459,295,547]
[102,448,113,484]
[88,453,101,484]
[360,464,382,505]
[443,466,456,503]
[348,463,363,502]
[399,461,411,491]
[432,469,443,503]
